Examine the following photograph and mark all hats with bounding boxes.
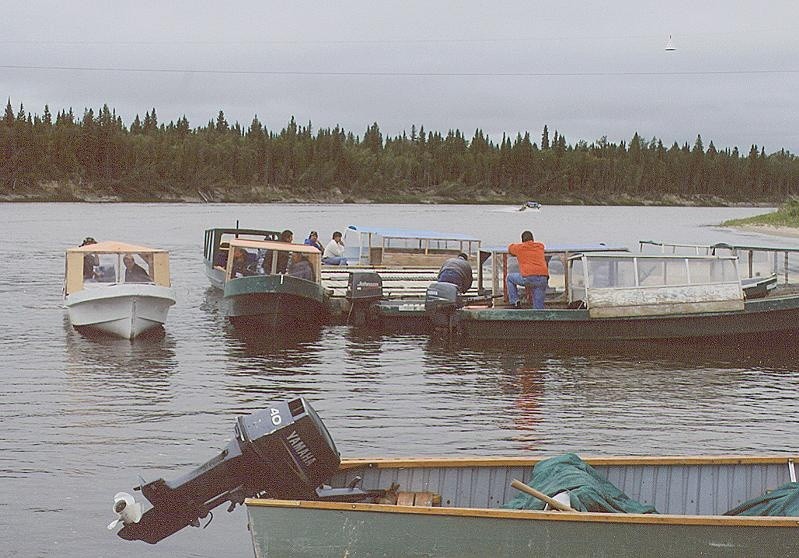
[310,231,318,235]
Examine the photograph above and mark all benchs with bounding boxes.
[395,492,434,508]
[545,473,571,514]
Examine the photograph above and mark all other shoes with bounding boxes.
[504,300,522,309]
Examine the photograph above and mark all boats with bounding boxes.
[62,236,177,342]
[202,219,799,355]
[107,395,799,558]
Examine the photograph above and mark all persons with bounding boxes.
[261,230,294,275]
[505,231,550,309]
[304,231,324,254]
[323,232,347,265]
[286,252,313,281]
[79,237,98,280]
[123,255,150,281]
[231,246,256,279]
[437,253,473,294]
